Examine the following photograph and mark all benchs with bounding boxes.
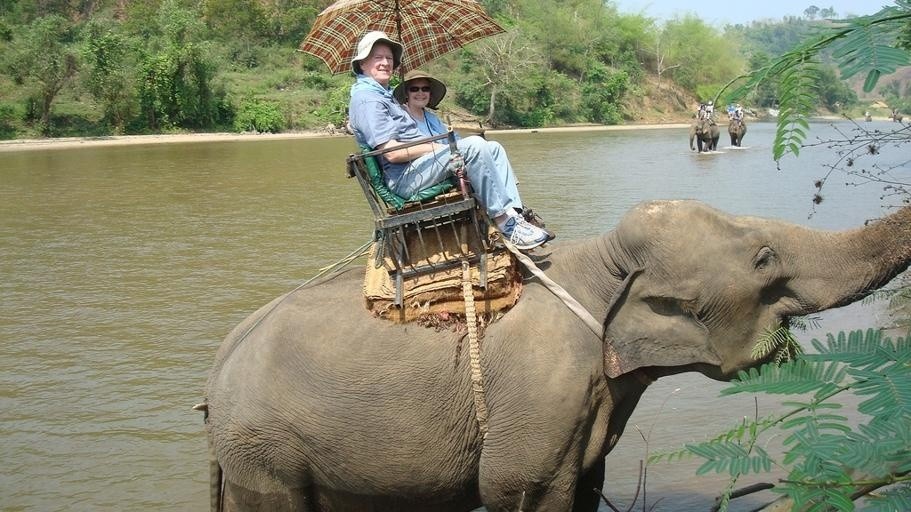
[345,117,504,310]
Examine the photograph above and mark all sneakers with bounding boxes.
[501,205,555,250]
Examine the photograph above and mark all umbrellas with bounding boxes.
[292,1,507,107]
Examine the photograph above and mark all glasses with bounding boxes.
[410,87,430,92]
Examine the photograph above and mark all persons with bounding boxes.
[349,27,552,254]
[694,99,745,127]
[387,67,557,246]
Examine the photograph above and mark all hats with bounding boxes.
[351,31,405,76]
[393,70,446,108]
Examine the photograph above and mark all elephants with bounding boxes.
[689,117,720,152]
[194,198,911,512]
[728,120,747,147]
